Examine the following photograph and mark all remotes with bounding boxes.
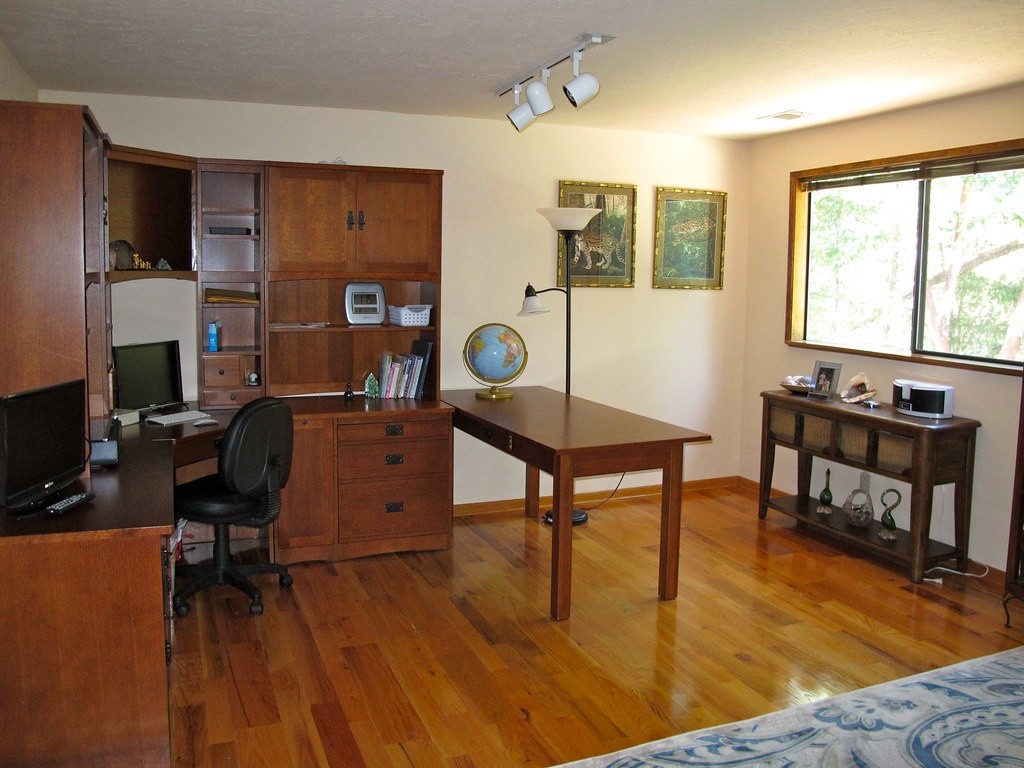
[44,492,96,517]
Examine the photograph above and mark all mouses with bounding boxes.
[193,418,218,426]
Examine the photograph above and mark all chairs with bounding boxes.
[171,395,295,621]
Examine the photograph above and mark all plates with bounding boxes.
[110,240,135,269]
[779,383,814,393]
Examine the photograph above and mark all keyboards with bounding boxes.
[148,411,212,425]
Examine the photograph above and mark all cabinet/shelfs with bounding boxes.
[1,99,445,473]
[757,389,982,583]
[0,392,456,768]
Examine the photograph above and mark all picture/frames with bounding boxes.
[556,180,637,289]
[652,186,728,291]
[807,360,843,403]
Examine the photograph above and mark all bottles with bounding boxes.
[208,323,217,352]
[110,244,116,271]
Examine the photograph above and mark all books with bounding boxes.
[378,340,433,399]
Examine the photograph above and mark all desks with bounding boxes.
[439,385,713,621]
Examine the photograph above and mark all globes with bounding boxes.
[463,323,529,399]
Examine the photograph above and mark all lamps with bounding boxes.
[517,281,567,318]
[537,208,602,535]
[495,32,602,133]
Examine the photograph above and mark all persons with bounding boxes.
[818,371,830,391]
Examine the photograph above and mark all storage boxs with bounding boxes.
[892,377,954,419]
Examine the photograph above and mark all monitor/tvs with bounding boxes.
[0,378,86,520]
[112,339,183,420]
